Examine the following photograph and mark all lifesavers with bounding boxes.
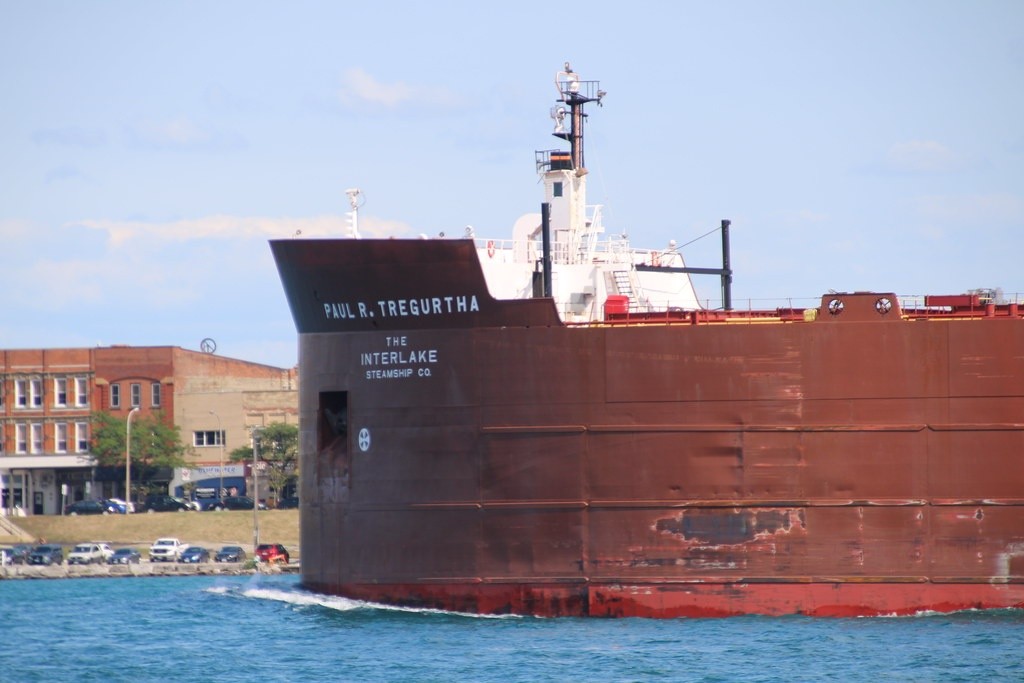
[487,240,496,257]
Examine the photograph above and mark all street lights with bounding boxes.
[125,406,140,515]
[208,410,223,508]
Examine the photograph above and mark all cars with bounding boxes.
[97,543,114,561]
[11,544,32,564]
[252,541,289,565]
[276,495,299,511]
[108,498,135,513]
[210,495,268,511]
[64,497,126,516]
[214,544,245,562]
[108,549,143,567]
[179,546,210,566]
[142,495,193,513]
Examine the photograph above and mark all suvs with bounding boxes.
[66,542,103,565]
[26,543,62,567]
[149,538,181,563]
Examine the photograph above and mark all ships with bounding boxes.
[265,62,1024,630]
